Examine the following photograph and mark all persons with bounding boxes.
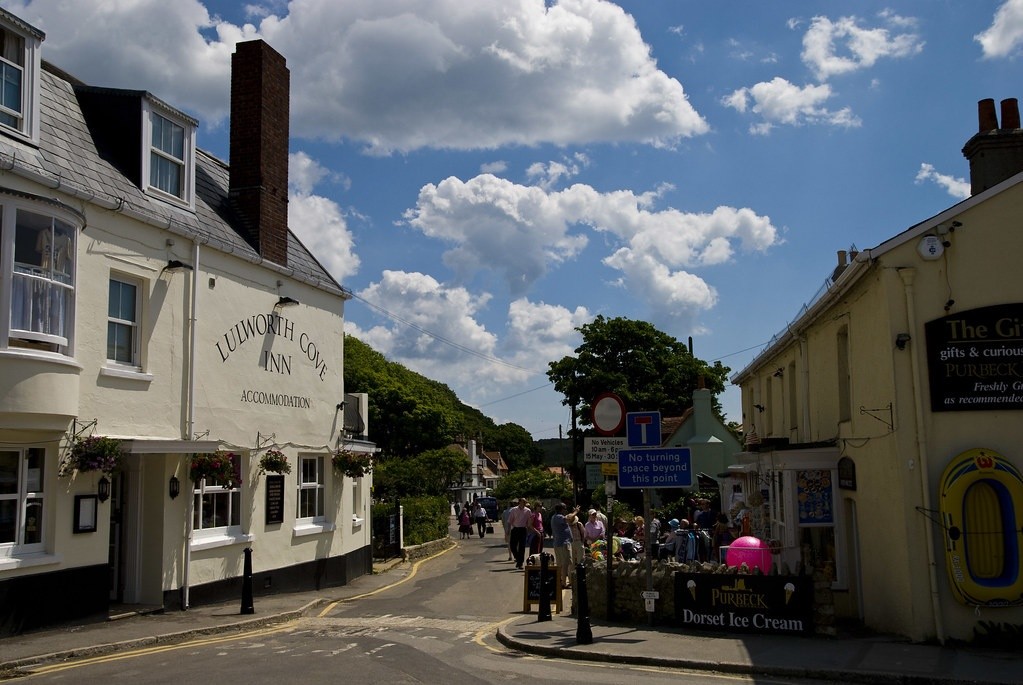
[502,498,545,570]
[551,499,716,589]
[454,500,500,539]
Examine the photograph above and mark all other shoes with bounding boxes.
[562,583,572,589]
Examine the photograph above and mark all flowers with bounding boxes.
[331,449,372,477]
[58,437,130,477]
[190,451,242,490]
[257,450,292,476]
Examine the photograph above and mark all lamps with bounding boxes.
[98,475,111,503]
[170,475,180,499]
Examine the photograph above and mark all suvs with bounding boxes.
[470,496,499,523]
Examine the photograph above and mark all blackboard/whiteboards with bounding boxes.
[524,565,561,604]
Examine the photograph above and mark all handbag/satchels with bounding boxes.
[577,523,586,543]
[469,526,473,535]
[486,526,494,533]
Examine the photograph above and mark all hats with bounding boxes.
[589,509,597,515]
[566,514,579,525]
[668,518,679,527]
